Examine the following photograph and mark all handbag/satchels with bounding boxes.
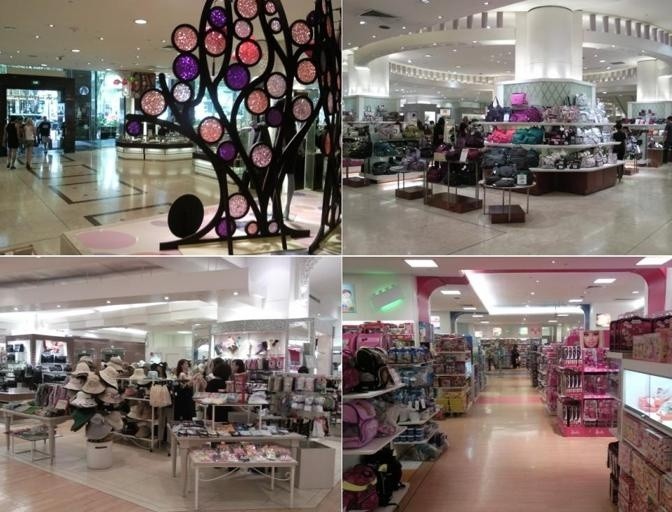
[41,355,72,381]
[7,345,23,360]
[342,92,611,187]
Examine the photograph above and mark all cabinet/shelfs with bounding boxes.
[0,333,342,512]
[97,119,256,187]
[341,315,672,510]
[344,91,672,226]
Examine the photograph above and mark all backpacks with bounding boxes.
[343,331,405,512]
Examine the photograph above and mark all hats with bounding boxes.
[64,356,159,440]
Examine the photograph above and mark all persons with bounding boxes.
[298,366,309,373]
[431,118,446,170]
[511,344,522,369]
[584,331,599,348]
[3,112,52,170]
[613,122,627,181]
[206,356,253,473]
[44,339,63,352]
[178,355,189,380]
[662,115,672,164]
